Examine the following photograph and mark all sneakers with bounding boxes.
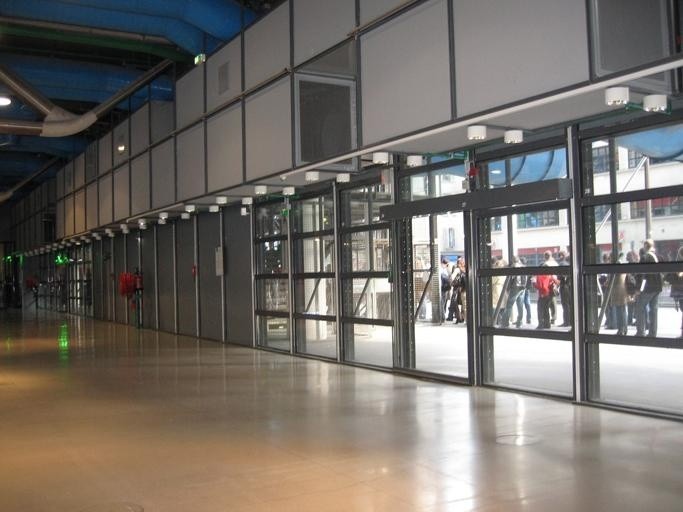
[446,315,661,338]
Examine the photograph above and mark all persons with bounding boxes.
[416,255,467,325]
[597,239,663,338]
[490,250,572,330]
[672,247,683,311]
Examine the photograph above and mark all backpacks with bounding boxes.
[441,275,451,292]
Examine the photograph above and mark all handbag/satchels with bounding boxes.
[550,281,558,296]
[625,275,637,294]
